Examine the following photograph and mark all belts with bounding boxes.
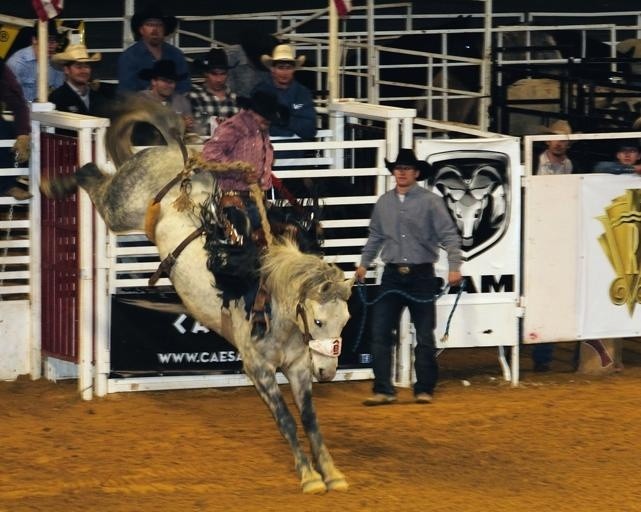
[385,264,432,274]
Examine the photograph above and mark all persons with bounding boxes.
[354,147,462,406]
[200,89,321,299]
[594,140,640,173]
[527,120,576,370]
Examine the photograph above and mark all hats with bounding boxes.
[50,44,103,66]
[259,43,307,70]
[33,18,70,38]
[235,87,292,129]
[383,146,433,182]
[136,59,182,83]
[549,118,573,134]
[129,0,181,33]
[189,47,239,74]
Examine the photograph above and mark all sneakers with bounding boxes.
[362,392,395,405]
[415,392,435,403]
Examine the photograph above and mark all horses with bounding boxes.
[39,90,361,496]
